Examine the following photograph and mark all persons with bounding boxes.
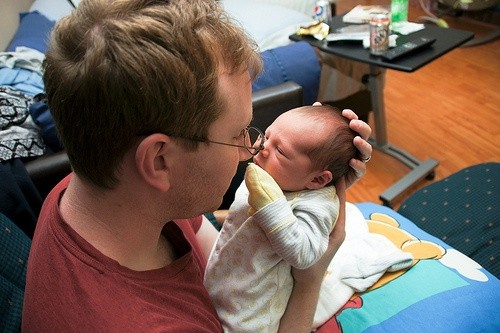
[20,0,372,333]
[203,105,361,333]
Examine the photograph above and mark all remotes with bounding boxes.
[382,36,435,61]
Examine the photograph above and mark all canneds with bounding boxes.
[391,0,409,22]
[369,14,390,55]
[315,0,333,32]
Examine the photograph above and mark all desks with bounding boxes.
[288,11,474,205]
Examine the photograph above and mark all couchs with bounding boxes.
[1,0,366,191]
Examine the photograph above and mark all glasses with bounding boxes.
[164,126,265,154]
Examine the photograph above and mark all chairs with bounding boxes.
[0,163,500,333]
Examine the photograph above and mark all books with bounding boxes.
[343,5,389,23]
[326,24,371,40]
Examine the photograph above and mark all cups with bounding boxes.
[391,0,409,29]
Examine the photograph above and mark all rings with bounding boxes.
[362,156,371,163]
